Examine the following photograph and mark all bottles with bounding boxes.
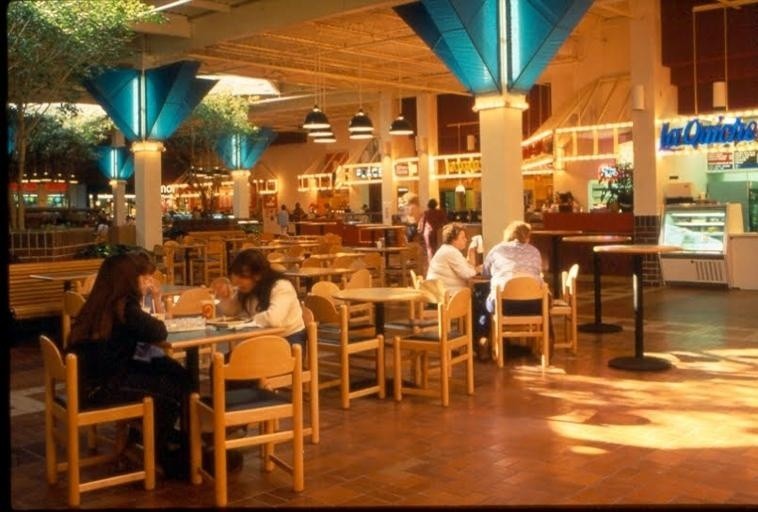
[199,300,216,318]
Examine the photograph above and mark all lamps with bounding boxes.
[308,54,334,137]
[388,69,414,136]
[350,132,374,140]
[313,63,336,143]
[303,51,331,129]
[347,62,374,132]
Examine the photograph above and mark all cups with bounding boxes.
[150,313,165,334]
[202,300,216,320]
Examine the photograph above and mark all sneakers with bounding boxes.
[117,449,136,470]
[140,452,162,479]
[478,337,488,360]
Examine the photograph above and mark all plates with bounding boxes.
[206,315,253,328]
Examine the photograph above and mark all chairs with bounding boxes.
[490,276,548,367]
[341,270,374,358]
[302,297,386,408]
[271,263,286,273]
[75,274,97,296]
[189,240,223,285]
[394,288,474,406]
[410,269,465,354]
[551,271,568,343]
[182,235,193,244]
[267,252,285,265]
[295,258,323,294]
[320,232,342,254]
[62,290,97,450]
[163,241,186,285]
[550,277,578,354]
[153,245,174,285]
[288,245,305,262]
[166,288,216,360]
[9,259,106,319]
[190,335,304,506]
[39,335,155,506]
[385,280,451,385]
[200,277,235,352]
[329,245,344,252]
[363,251,385,287]
[257,306,319,458]
[331,256,359,281]
[311,281,375,373]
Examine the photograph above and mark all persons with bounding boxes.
[403,197,420,242]
[475,220,554,363]
[278,204,289,235]
[293,202,304,234]
[418,199,449,262]
[64,254,185,479]
[425,222,478,311]
[362,203,371,214]
[208,249,306,390]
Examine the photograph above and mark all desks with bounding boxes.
[530,230,583,299]
[562,235,632,333]
[593,244,684,371]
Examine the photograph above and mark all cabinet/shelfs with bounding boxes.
[657,203,744,287]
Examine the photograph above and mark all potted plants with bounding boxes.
[598,162,633,214]
